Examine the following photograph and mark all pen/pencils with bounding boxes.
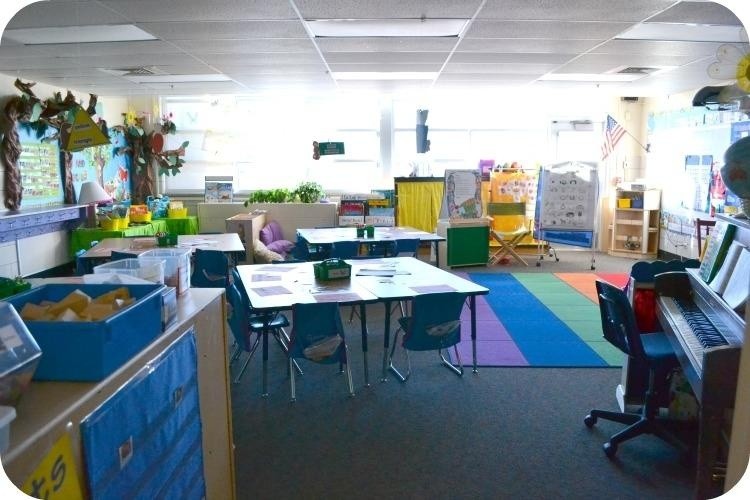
[356,224,365,228]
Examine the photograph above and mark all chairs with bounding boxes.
[326,240,359,260]
[191,247,304,383]
[388,292,467,382]
[390,239,419,257]
[483,201,533,270]
[287,304,356,400]
[696,216,718,259]
[584,273,683,466]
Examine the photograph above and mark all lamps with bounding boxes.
[76,179,115,229]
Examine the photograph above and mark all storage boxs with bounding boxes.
[0,246,245,406]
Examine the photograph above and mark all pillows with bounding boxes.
[253,221,295,264]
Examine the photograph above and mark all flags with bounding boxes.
[599,114,628,161]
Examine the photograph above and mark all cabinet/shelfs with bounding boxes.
[606,187,663,261]
[224,213,265,264]
[0,276,238,500]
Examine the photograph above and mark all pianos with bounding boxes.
[654,212,750,500]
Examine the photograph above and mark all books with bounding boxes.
[710,240,750,311]
[696,221,739,283]
[338,194,397,227]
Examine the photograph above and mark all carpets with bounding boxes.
[444,269,632,369]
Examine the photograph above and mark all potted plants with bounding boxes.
[293,179,326,205]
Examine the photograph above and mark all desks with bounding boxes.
[297,225,448,267]
[76,232,245,287]
[236,256,490,397]
